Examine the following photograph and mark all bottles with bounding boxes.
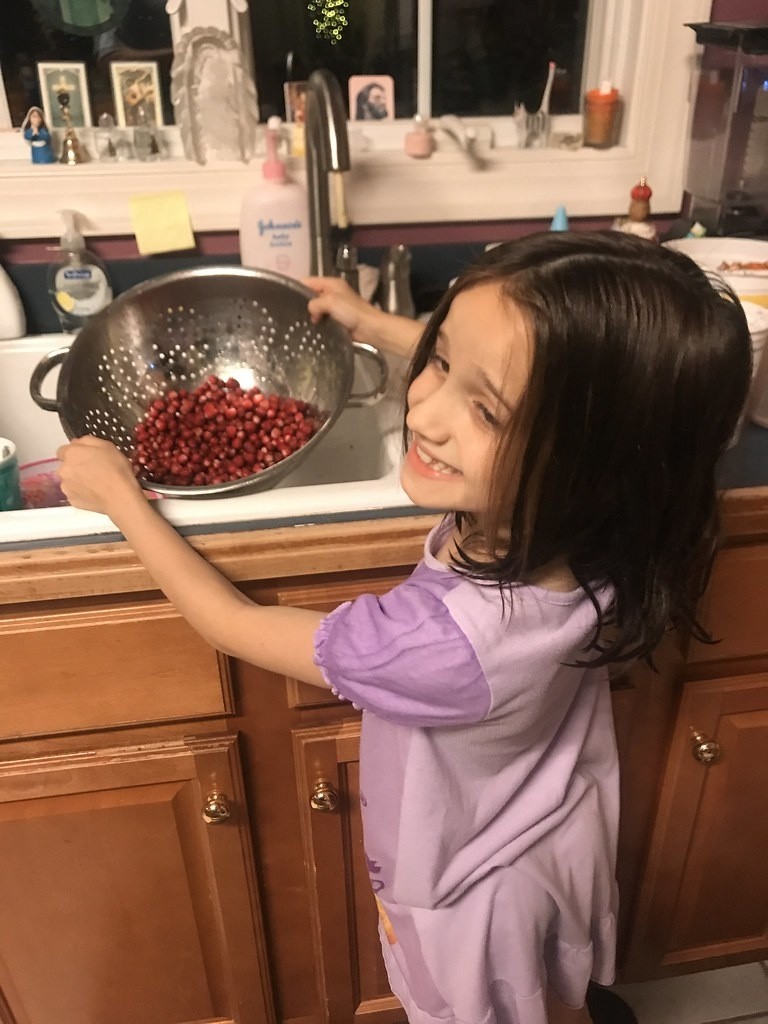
[0,436,24,511]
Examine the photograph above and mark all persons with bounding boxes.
[20,106,56,164]
[57,229,751,1024]
[356,84,388,120]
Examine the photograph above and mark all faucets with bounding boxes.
[306,71,352,279]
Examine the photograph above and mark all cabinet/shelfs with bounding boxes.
[1,569,646,1024]
[622,521,767,989]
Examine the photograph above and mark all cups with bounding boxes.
[661,236,768,448]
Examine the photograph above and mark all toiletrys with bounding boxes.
[54,216,112,329]
[242,123,305,293]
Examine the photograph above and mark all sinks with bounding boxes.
[2,327,426,545]
